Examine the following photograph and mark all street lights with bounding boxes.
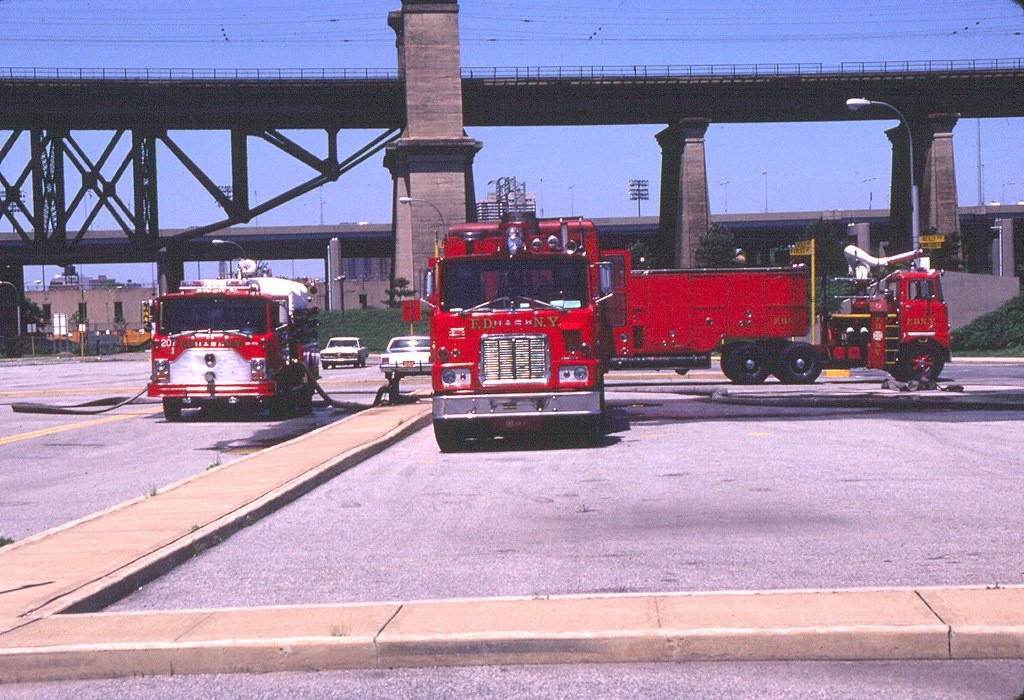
[212,239,247,260]
[0,281,21,336]
[399,196,445,228]
[846,97,920,267]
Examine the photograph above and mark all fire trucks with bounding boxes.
[141,275,321,422]
[600,241,952,386]
[419,210,619,456]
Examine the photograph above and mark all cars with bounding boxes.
[319,336,369,368]
[379,335,434,382]
